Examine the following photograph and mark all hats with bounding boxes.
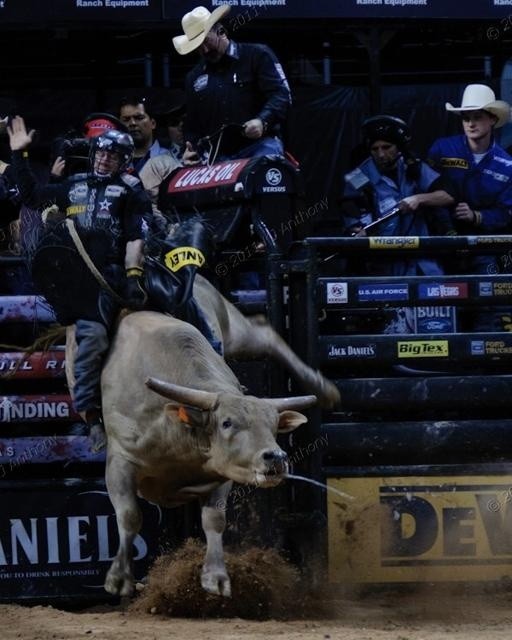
[81,112,129,139]
[443,83,511,130]
[362,114,413,152]
[172,4,231,57]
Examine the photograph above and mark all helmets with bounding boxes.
[88,130,136,184]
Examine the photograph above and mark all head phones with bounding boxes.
[215,24,226,36]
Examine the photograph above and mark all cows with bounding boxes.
[0,271,346,602]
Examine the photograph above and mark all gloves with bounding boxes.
[124,267,148,307]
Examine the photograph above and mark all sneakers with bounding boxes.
[89,423,108,455]
